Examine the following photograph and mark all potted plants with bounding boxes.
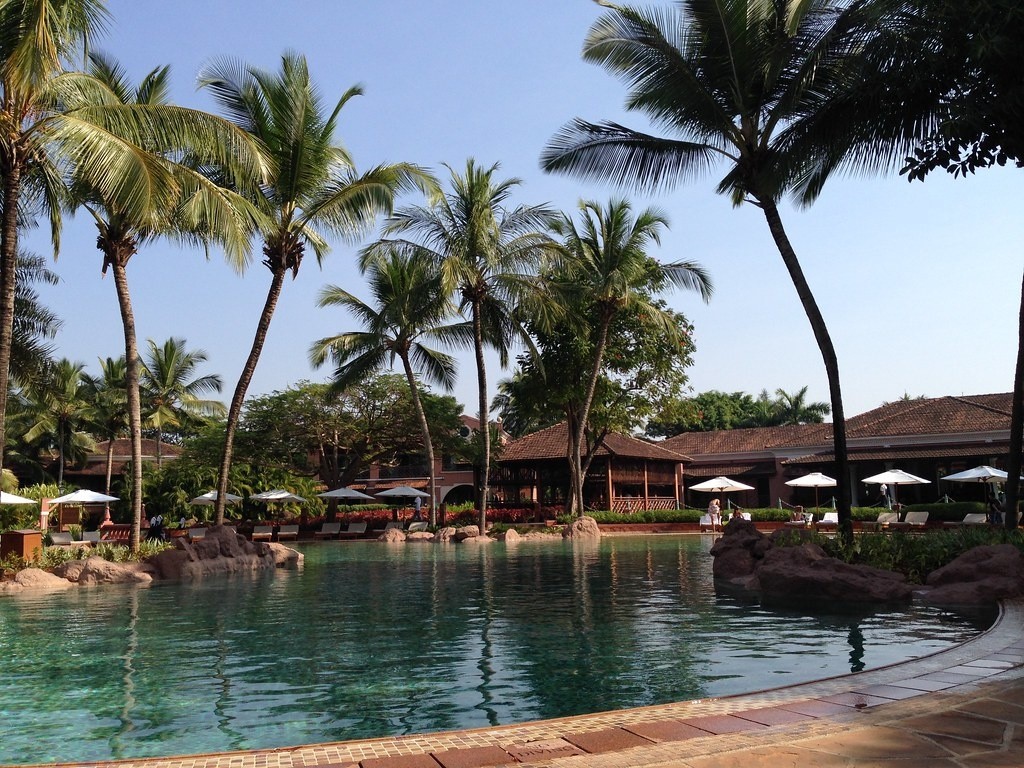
[543,505,565,526]
[67,524,82,541]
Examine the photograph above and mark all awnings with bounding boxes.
[781,442,1023,464]
[343,479,430,490]
[681,464,755,476]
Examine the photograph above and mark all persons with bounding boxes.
[733,508,741,520]
[413,496,421,520]
[708,499,720,532]
[790,512,805,521]
[988,492,1003,524]
[267,506,285,520]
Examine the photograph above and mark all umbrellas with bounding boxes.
[249,488,307,526]
[1,491,38,505]
[940,465,1024,521]
[47,487,121,541]
[315,488,375,513]
[861,469,932,522]
[784,472,837,531]
[689,476,755,520]
[188,488,243,505]
[374,484,431,520]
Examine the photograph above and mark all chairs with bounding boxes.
[943,513,990,527]
[728,512,752,521]
[50,532,93,549]
[784,513,814,529]
[1000,512,1023,526]
[405,522,428,534]
[816,512,838,532]
[889,511,929,534]
[252,526,274,542]
[83,532,117,547]
[862,512,901,532]
[373,521,404,533]
[276,525,299,542]
[340,523,367,540]
[699,514,723,533]
[189,528,208,544]
[314,522,341,540]
